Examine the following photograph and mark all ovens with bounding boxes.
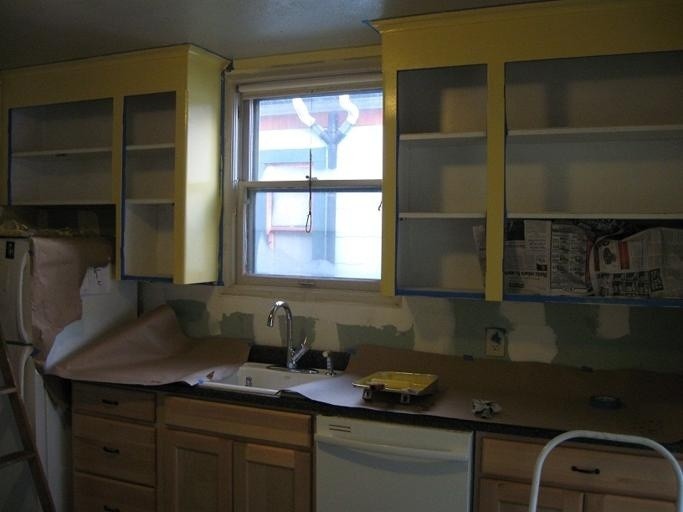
[312,412,473,511]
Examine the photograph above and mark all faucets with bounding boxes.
[266,298,309,367]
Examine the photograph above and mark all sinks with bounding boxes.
[196,360,346,399]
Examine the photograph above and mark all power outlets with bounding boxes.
[485,329,506,356]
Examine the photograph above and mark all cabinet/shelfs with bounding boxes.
[474,432,683,511]
[363,2,683,307]
[69,381,156,512]
[156,396,315,512]
[1,42,233,288]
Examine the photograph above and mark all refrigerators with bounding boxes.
[0,234,140,512]
